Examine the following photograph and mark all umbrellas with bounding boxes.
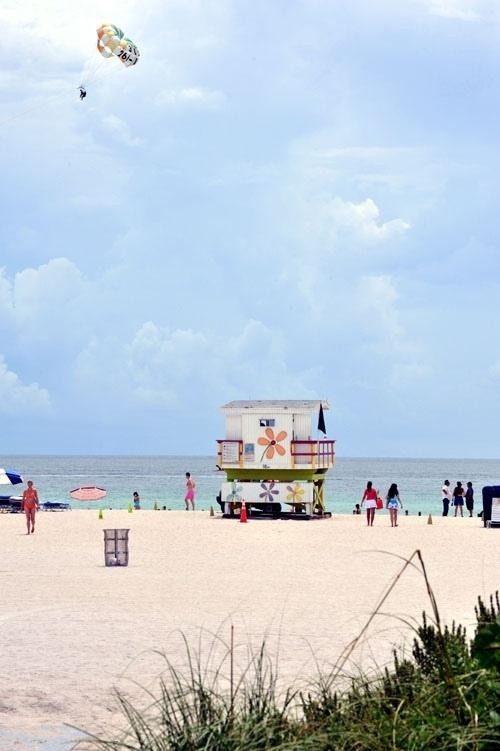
[71,485,108,510]
[0,465,23,484]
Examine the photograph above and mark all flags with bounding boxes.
[319,404,326,433]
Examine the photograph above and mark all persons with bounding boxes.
[464,482,474,517]
[80,89,86,99]
[133,492,140,510]
[453,482,465,517]
[441,479,451,516]
[359,481,380,526]
[21,480,40,535]
[353,504,360,514]
[184,472,195,510]
[384,483,402,527]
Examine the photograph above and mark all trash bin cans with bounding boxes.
[102,529,130,566]
